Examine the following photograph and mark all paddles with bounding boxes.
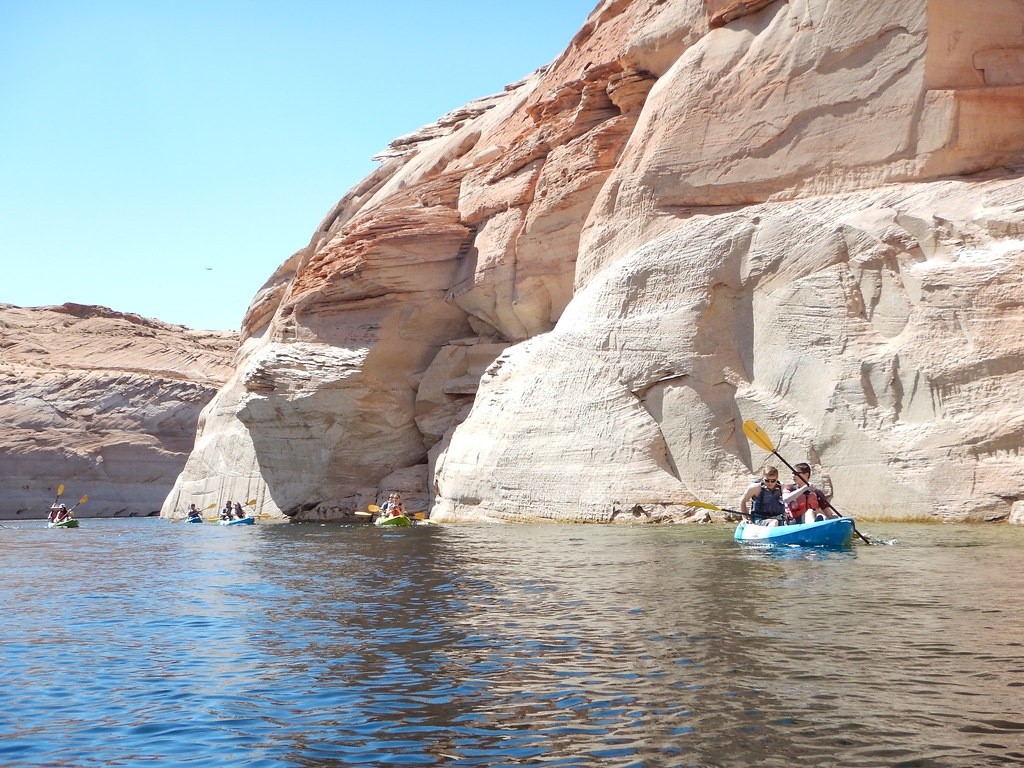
[682,500,776,521]
[741,419,873,545]
[368,505,388,512]
[405,512,425,521]
[354,512,383,517]
[238,499,257,508]
[208,513,271,523]
[171,502,216,524]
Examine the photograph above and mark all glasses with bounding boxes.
[791,470,803,475]
[763,477,777,483]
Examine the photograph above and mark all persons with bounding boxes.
[53,503,74,523]
[231,501,246,520]
[738,465,786,527]
[48,502,60,524]
[219,500,234,521]
[385,494,405,518]
[379,493,394,519]
[781,462,838,525]
[187,503,203,519]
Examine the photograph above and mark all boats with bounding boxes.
[215,515,255,527]
[375,515,412,527]
[734,517,856,548]
[48,518,80,528]
[184,517,204,523]
